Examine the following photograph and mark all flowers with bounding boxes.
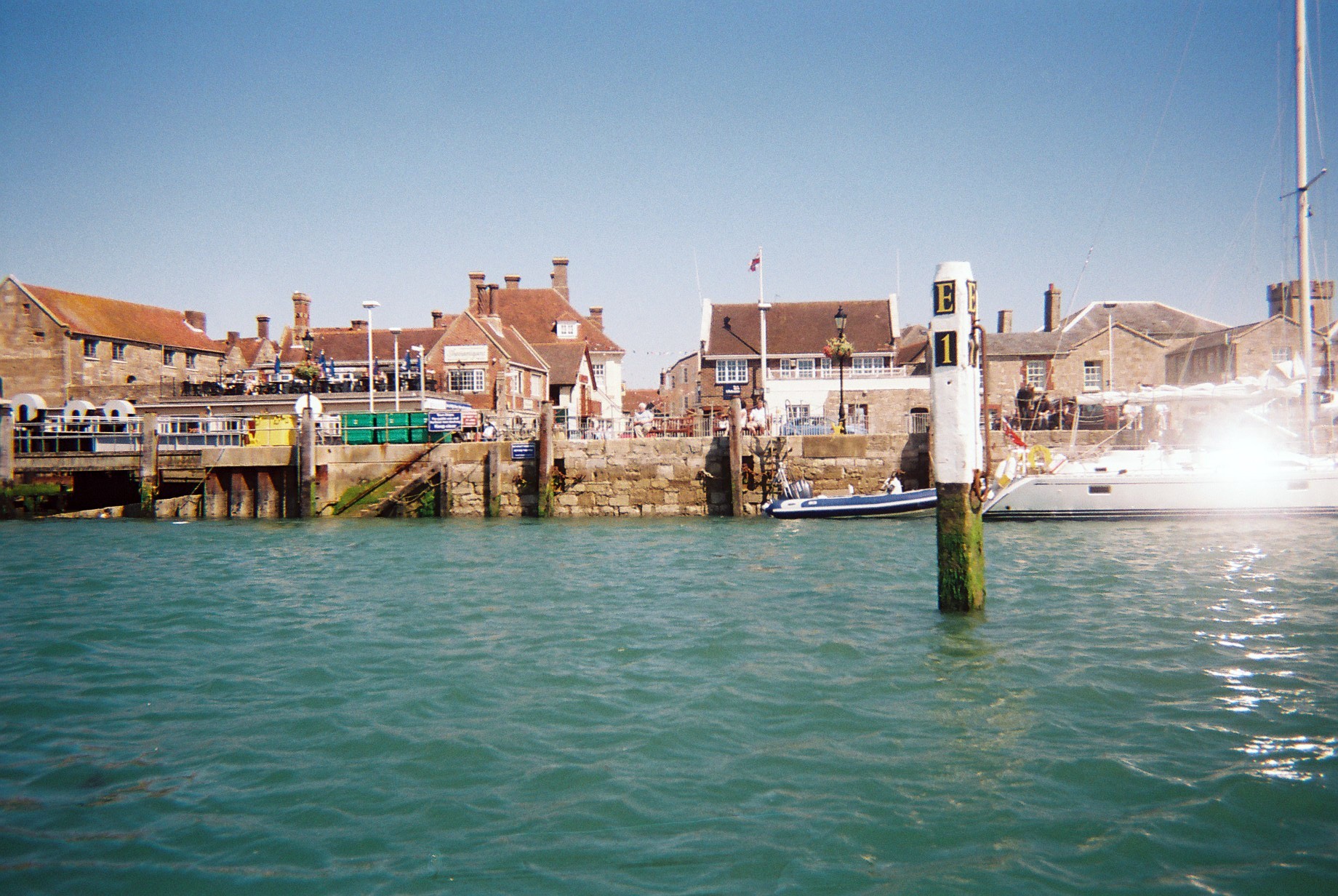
[291,360,322,381]
[822,335,854,361]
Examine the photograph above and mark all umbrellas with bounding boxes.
[404,350,411,370]
[416,354,421,372]
[274,355,281,380]
[318,349,327,377]
[329,357,336,377]
[374,356,379,375]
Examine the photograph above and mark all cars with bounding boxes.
[778,416,866,435]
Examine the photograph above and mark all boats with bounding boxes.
[976,220,1338,526]
[760,432,939,519]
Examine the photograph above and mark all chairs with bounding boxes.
[182,376,436,398]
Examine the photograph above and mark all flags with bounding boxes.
[747,253,760,273]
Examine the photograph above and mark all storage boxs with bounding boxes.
[319,412,452,446]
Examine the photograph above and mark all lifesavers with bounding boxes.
[1028,445,1051,467]
[994,457,1016,488]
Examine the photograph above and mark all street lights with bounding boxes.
[362,299,381,412]
[410,345,426,411]
[218,357,225,387]
[302,329,315,408]
[389,327,401,412]
[833,306,850,434]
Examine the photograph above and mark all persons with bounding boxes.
[723,400,764,437]
[1121,400,1144,431]
[1016,381,1069,431]
[483,421,494,439]
[879,471,902,494]
[633,402,654,437]
[180,368,394,396]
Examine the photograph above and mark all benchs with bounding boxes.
[635,416,693,437]
[714,413,773,436]
